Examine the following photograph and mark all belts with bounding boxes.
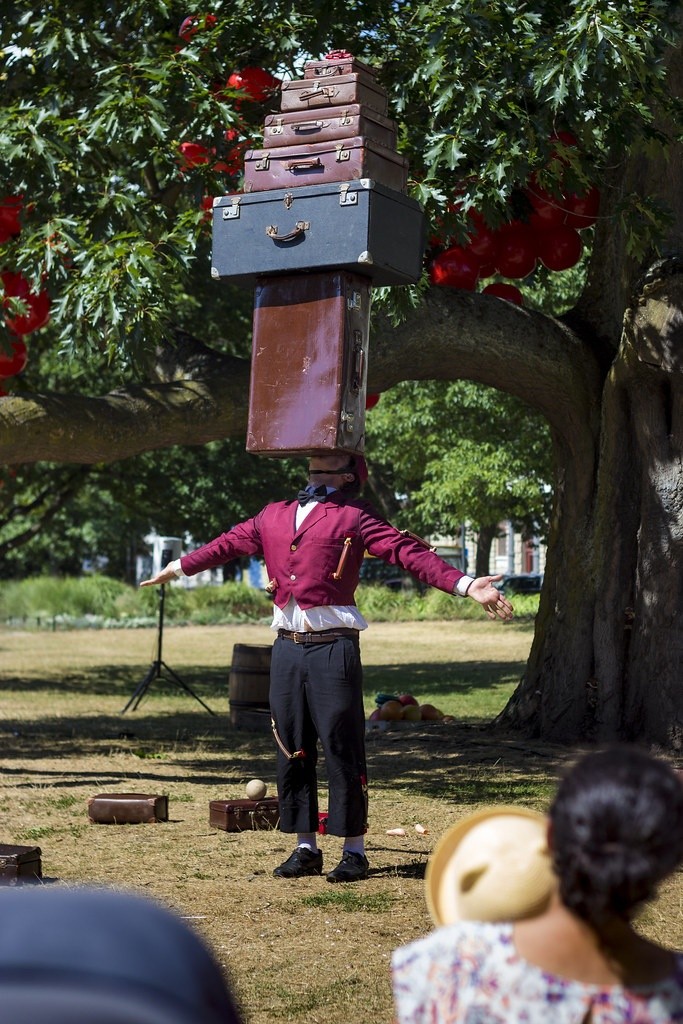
[276,628,359,642]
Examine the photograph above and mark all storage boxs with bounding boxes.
[0,844,42,887]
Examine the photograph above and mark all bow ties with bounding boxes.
[297,483,328,505]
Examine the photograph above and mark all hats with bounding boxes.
[425,804,554,929]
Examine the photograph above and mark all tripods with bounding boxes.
[121,583,218,720]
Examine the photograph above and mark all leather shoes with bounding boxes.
[326,849,369,882]
[272,847,324,877]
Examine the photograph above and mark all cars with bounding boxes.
[490,575,543,598]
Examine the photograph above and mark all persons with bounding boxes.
[139,451,516,884]
[392,749,683,1024]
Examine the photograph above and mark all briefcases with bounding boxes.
[209,795,281,830]
[264,102,398,153]
[210,178,426,290]
[303,49,379,85]
[88,792,170,824]
[243,266,372,458]
[243,134,409,195]
[280,72,389,117]
[0,843,44,887]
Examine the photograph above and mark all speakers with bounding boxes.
[153,536,182,576]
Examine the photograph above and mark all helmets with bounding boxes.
[347,453,369,502]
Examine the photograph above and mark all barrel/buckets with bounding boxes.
[229,644,273,728]
